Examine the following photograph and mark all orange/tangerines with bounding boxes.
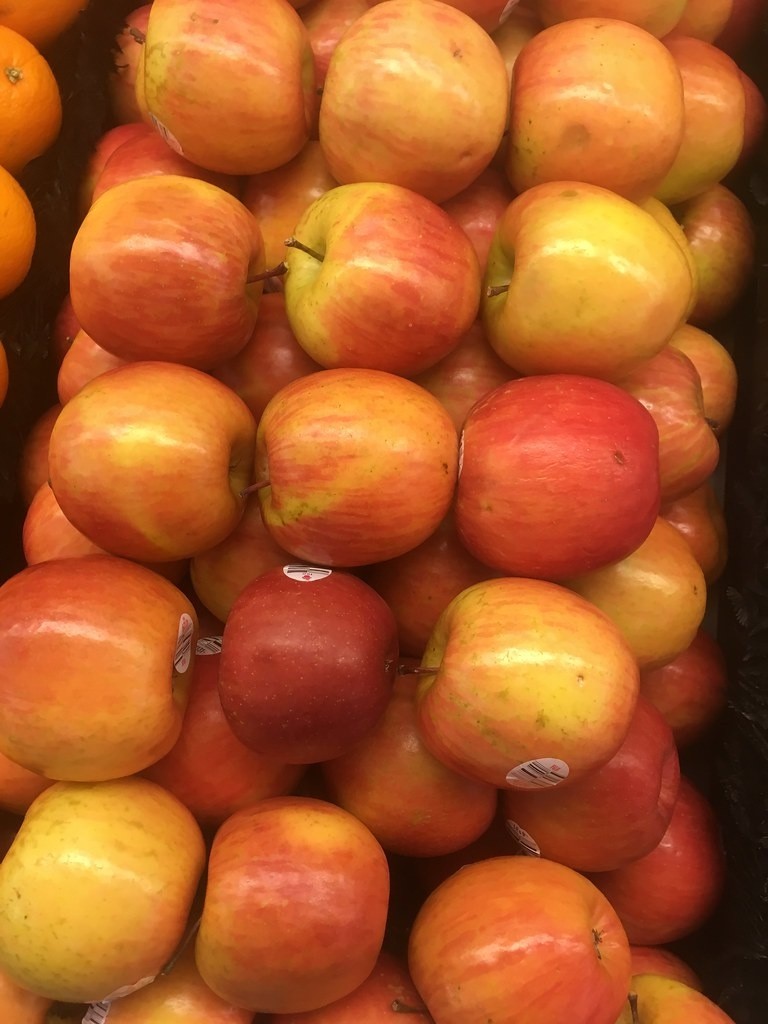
[0,0,90,49]
[0,165,37,299]
[0,25,63,174]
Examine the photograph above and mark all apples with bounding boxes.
[0,0,768,1024]
